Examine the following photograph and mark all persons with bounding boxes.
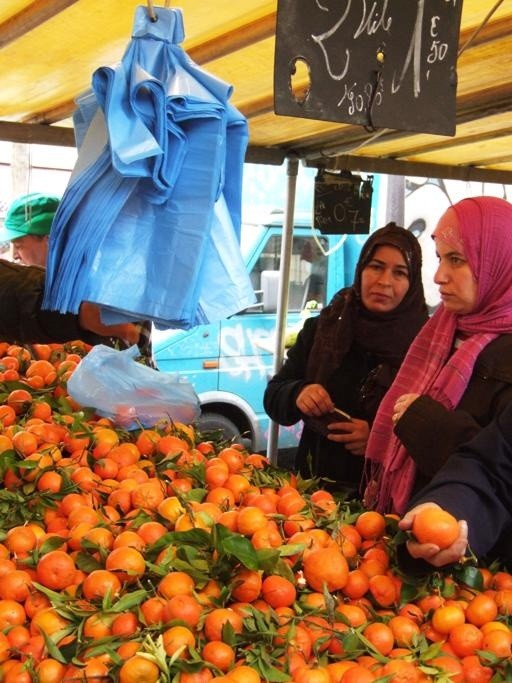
[357,196,512,574]
[263,221,432,501]
[0,192,61,266]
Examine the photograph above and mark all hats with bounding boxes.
[0,192,61,242]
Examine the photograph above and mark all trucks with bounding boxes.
[108,203,388,474]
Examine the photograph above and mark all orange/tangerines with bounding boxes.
[0,340,512,683]
